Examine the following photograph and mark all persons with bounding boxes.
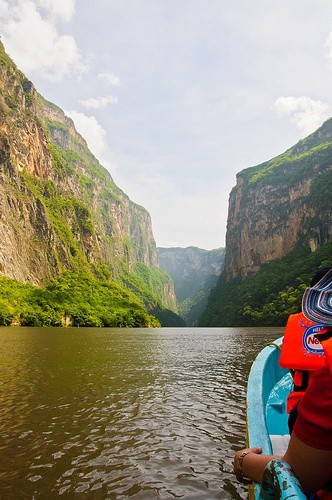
[232,266,332,500]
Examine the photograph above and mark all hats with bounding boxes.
[301,268,332,326]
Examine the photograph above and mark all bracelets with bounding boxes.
[237,452,250,477]
